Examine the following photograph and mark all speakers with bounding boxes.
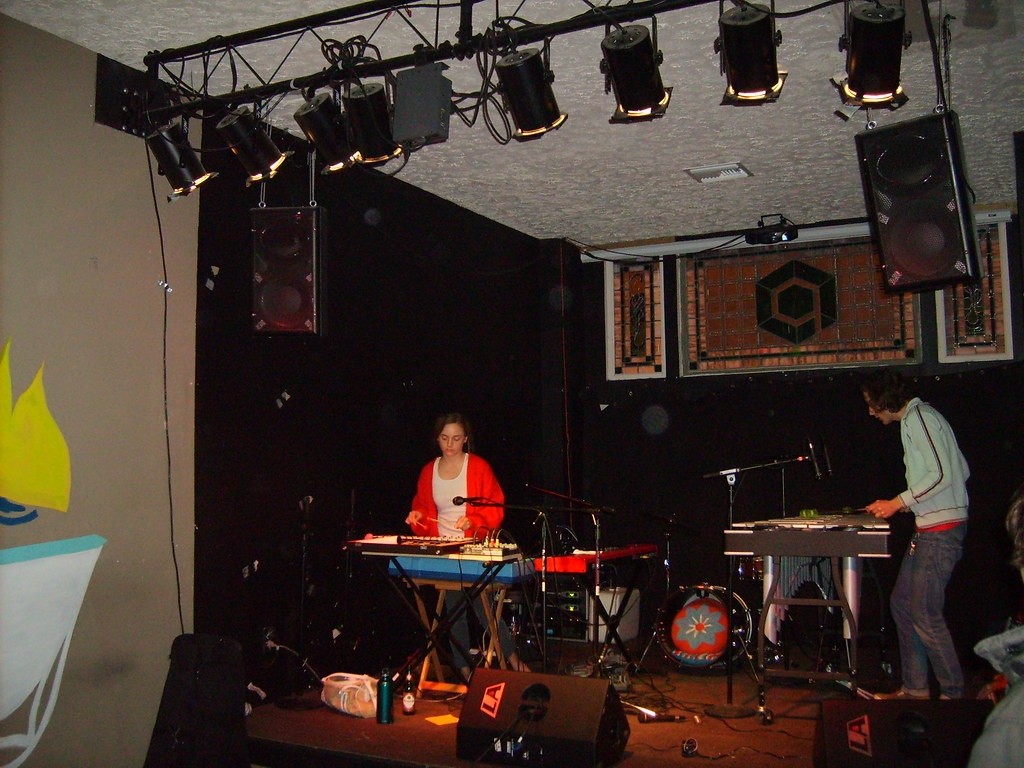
[812,699,995,768]
[853,110,985,295]
[247,206,327,339]
[456,668,631,768]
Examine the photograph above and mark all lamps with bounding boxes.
[714,0,789,107]
[495,38,568,143]
[599,16,674,123]
[216,102,295,187]
[144,112,219,204]
[829,0,912,121]
[293,71,404,173]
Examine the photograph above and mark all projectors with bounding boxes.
[745,225,798,244]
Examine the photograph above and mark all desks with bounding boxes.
[403,577,509,698]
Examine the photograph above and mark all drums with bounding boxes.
[738,554,765,583]
[656,582,754,670]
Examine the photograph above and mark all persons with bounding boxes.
[405,409,531,682]
[967,492,1024,768]
[861,367,971,714]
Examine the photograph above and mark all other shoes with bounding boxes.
[873,691,931,700]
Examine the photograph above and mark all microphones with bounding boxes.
[807,438,822,479]
[453,497,479,505]
[637,712,686,723]
[822,439,834,477]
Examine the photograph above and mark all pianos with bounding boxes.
[535,544,660,678]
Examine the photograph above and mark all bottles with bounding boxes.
[403,665,416,714]
[377,668,392,723]
